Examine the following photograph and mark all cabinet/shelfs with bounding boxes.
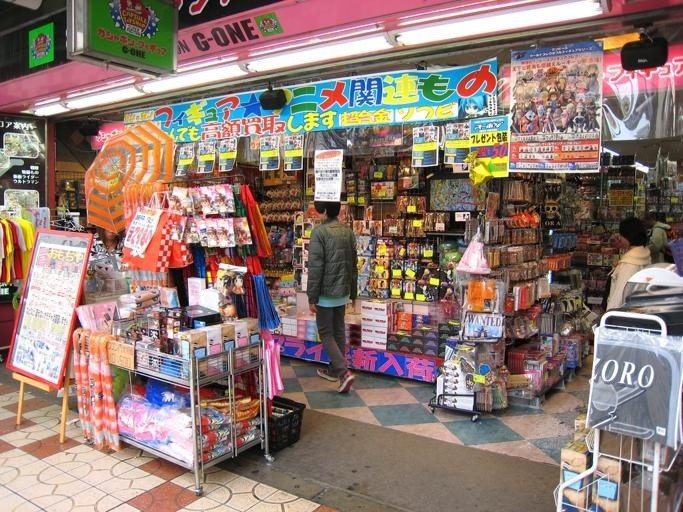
[69,299,275,496]
[58,150,682,401]
[549,259,683,512]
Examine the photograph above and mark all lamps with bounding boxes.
[257,80,287,109]
[619,21,668,71]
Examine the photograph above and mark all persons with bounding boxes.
[307,201,358,393]
[606,212,667,312]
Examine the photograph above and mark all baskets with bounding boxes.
[265,395,307,453]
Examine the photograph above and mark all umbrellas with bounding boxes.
[73,328,120,452]
[84,122,174,234]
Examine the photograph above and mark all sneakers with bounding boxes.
[316,367,338,382]
[336,370,356,395]
